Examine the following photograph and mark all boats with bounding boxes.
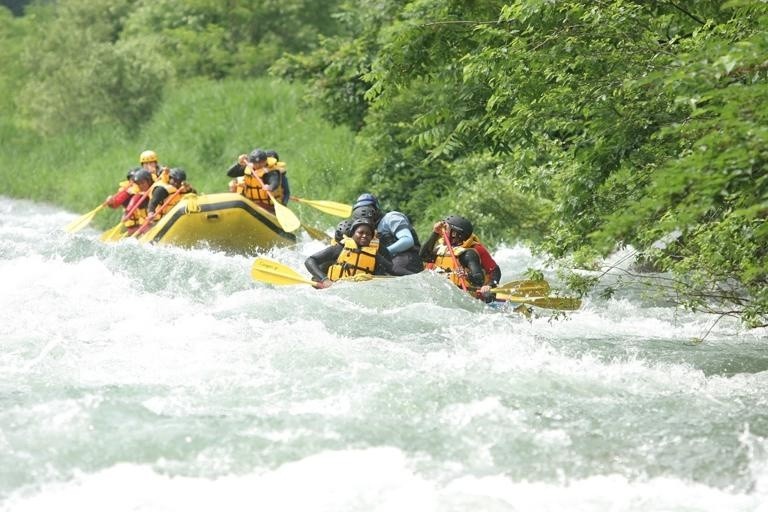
[135,193,332,257]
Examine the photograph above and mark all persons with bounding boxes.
[106,151,197,235]
[305,192,501,303]
[228,149,289,214]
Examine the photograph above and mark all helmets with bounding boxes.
[126,150,186,189]
[443,215,473,242]
[334,193,379,243]
[246,148,278,167]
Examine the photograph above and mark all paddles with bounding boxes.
[243,158,300,233]
[251,258,325,287]
[290,197,352,219]
[457,279,582,311]
[300,222,333,246]
[66,172,186,243]
